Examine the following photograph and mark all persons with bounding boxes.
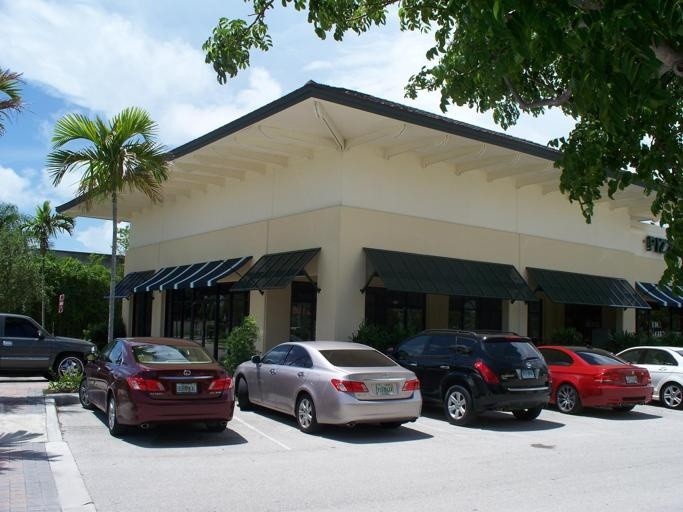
[89,321,105,350]
[113,315,127,339]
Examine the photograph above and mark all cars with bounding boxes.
[535,345,655,417]
[79,335,236,438]
[231,340,424,433]
[610,346,683,409]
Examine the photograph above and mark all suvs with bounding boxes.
[385,324,554,426]
[1,312,98,390]
